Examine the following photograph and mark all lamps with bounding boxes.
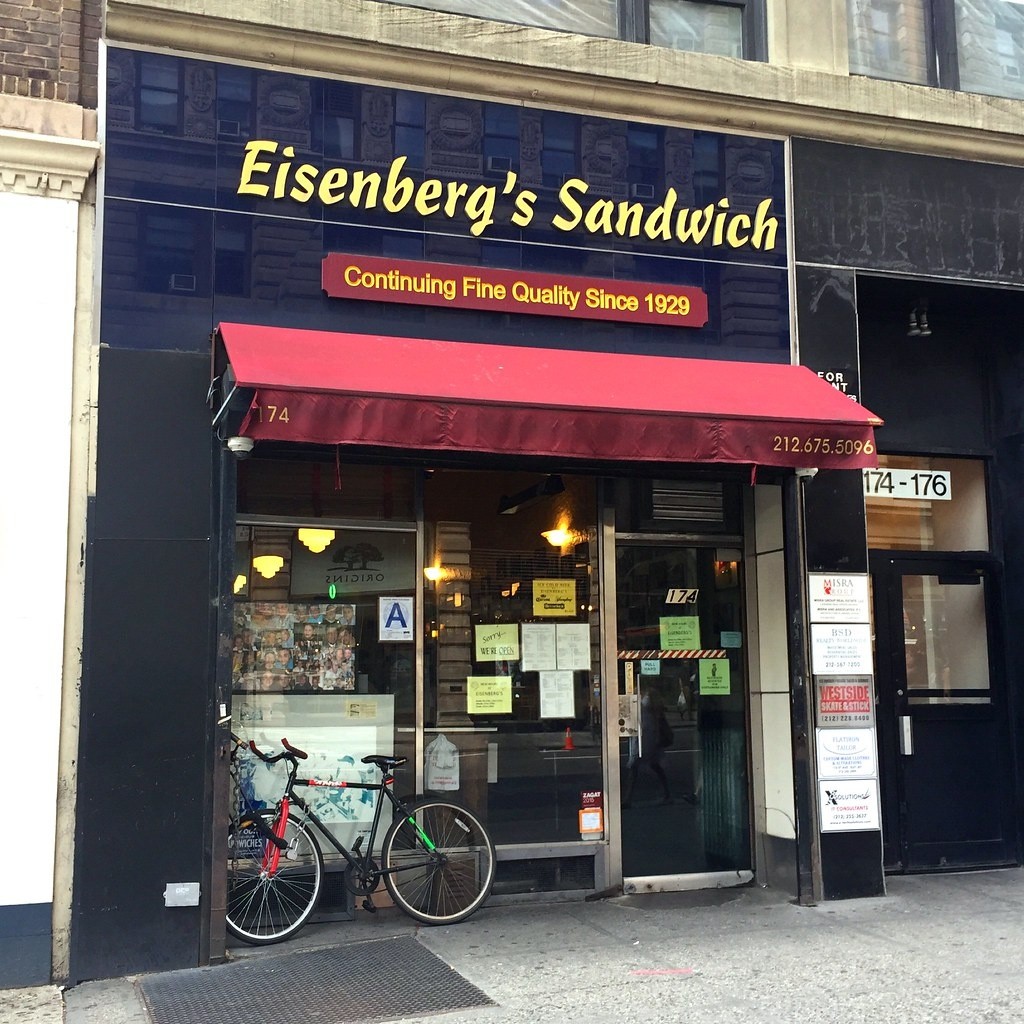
[541,530,574,546]
[298,528,336,554]
[253,555,285,579]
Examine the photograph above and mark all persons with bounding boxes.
[620,679,702,810]
[230,602,356,690]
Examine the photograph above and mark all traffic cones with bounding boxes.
[562,726,576,750]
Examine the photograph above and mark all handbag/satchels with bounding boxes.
[676,691,687,712]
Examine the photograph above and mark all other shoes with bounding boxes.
[683,792,699,806]
[658,791,673,805]
[689,718,696,721]
[621,800,632,809]
[681,717,684,720]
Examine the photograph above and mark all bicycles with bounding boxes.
[225,738,498,946]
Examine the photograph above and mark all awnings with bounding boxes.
[211,320,883,470]
